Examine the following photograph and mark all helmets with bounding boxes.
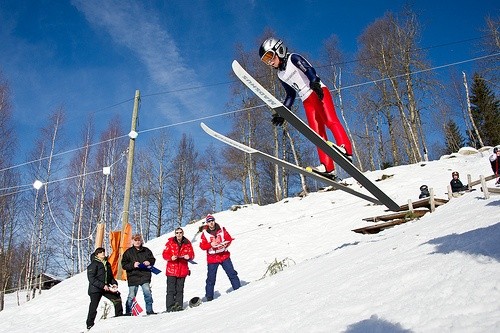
[493,146,500,154]
[452,172,459,178]
[258,37,288,70]
[420,185,429,191]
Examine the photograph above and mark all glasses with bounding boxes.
[175,231,182,234]
[261,50,275,66]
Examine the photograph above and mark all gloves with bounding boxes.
[310,82,324,100]
[271,106,291,126]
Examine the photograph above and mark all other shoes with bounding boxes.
[147,312,157,315]
[87,325,93,329]
[167,308,184,312]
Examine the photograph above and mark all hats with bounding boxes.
[132,234,142,241]
[205,215,215,223]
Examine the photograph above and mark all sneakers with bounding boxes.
[327,140,352,162]
[306,164,336,179]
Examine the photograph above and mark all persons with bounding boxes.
[419,185,430,200]
[258,36,353,180]
[450,171,469,192]
[121,234,158,316]
[199,213,241,302]
[162,227,195,312]
[85,247,125,330]
[489,147,500,185]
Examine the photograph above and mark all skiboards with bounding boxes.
[200,59,401,213]
